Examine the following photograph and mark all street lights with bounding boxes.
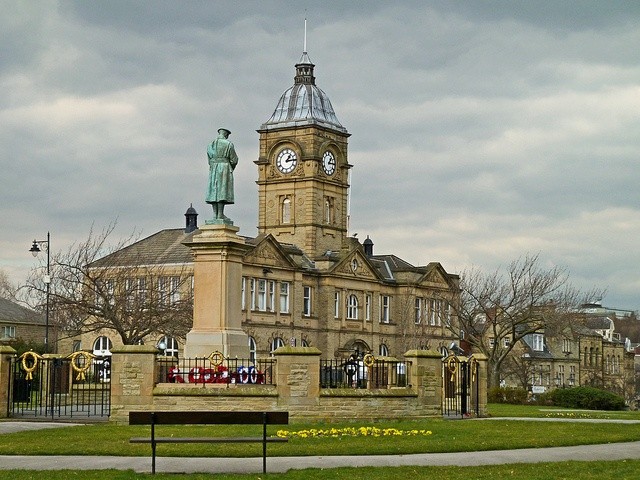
[29,231,50,354]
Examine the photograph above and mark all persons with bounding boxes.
[205,127,239,226]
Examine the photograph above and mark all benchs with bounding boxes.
[129,412,288,474]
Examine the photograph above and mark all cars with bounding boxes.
[342,356,406,389]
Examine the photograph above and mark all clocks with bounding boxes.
[275,146,298,175]
[351,260,359,271]
[322,149,337,176]
